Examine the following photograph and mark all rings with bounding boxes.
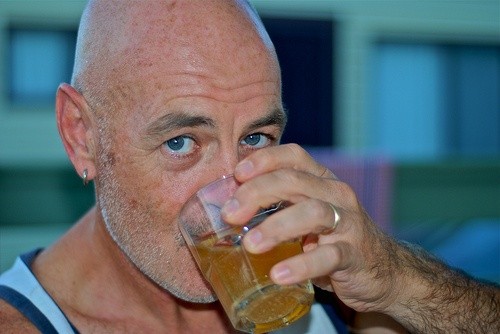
[322,203,339,234]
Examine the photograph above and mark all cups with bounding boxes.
[178,174,314,334]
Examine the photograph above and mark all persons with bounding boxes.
[1,0,500,334]
